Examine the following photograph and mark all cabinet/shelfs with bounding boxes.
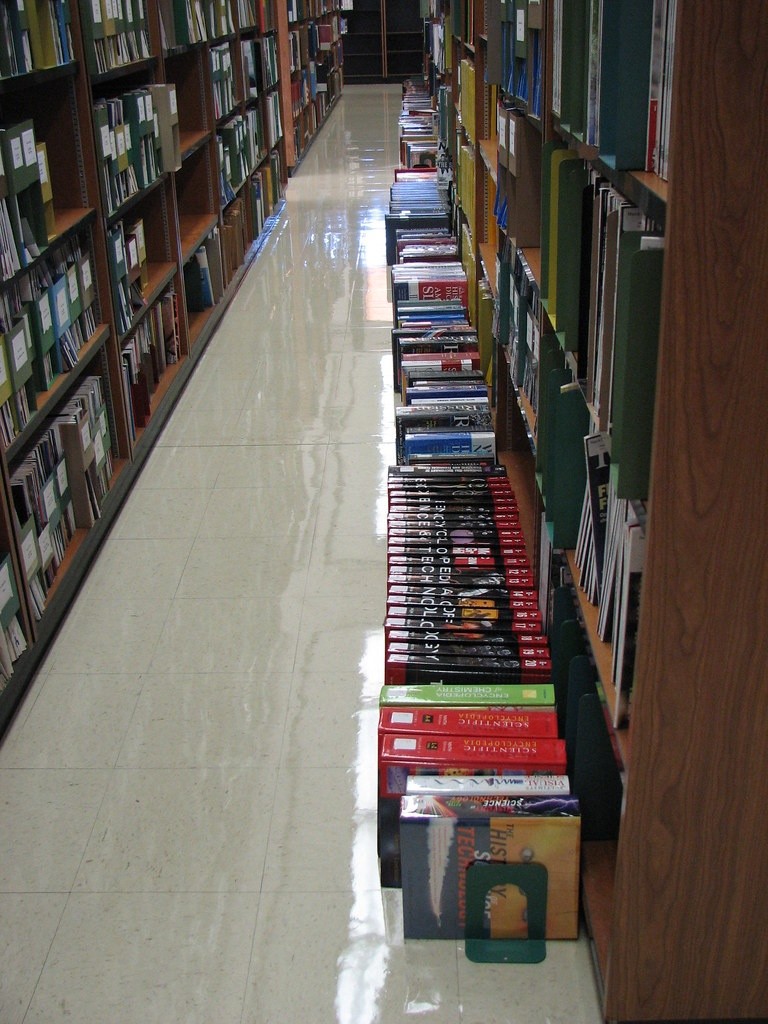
[342,0,424,84]
[0,0,344,745]
[433,0,768,1024]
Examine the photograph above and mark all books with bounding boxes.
[377,0,676,943]
[0,1,349,727]
